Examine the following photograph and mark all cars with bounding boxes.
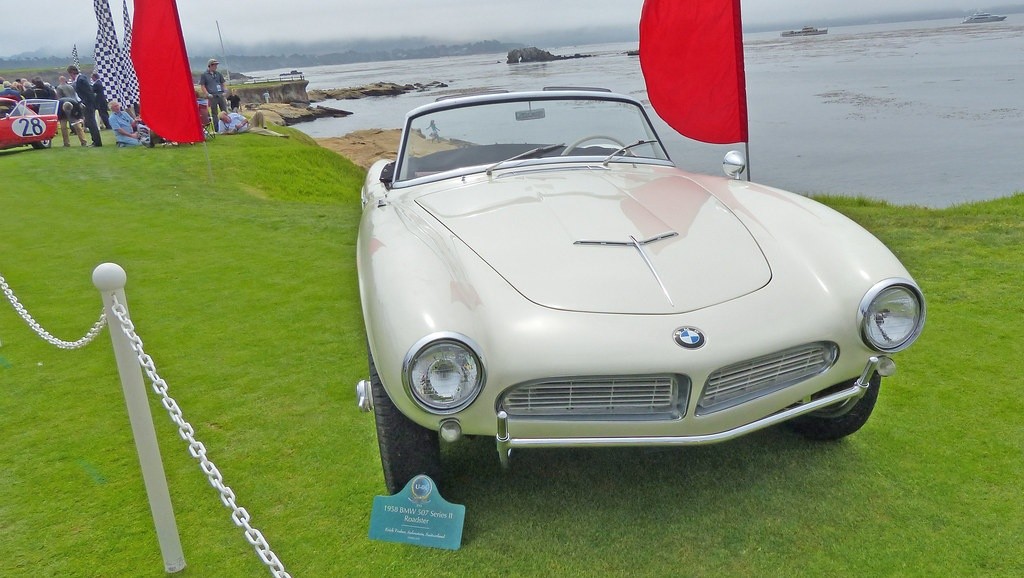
[0,95,61,150]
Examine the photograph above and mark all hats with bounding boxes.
[3,81,12,88]
[208,59,219,66]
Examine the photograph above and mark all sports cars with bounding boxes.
[353,84,931,501]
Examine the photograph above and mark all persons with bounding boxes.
[426,120,441,143]
[0,58,291,147]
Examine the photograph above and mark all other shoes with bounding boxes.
[81,140,87,146]
[283,134,290,138]
[86,142,96,147]
[65,143,70,147]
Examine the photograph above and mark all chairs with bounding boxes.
[202,117,216,141]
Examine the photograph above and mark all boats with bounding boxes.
[781,26,829,38]
[960,9,1008,24]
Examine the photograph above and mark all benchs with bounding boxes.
[381,145,624,187]
